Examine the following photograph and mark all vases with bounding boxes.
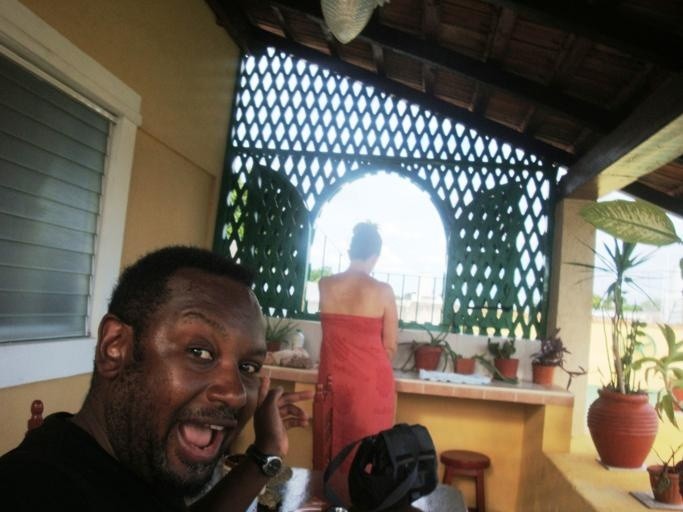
[531,363,555,384]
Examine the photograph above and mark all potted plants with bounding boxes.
[399,324,519,384]
[560,237,682,506]
[266,315,301,353]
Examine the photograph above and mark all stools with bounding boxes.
[440,448,490,512]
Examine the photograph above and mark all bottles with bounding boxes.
[291,328,305,349]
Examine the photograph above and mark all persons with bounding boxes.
[0,245,313,512]
[313,222,398,476]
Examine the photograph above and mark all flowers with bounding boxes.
[530,328,588,389]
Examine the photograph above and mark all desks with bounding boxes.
[186,467,467,512]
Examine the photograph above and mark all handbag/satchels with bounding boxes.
[322,422,439,512]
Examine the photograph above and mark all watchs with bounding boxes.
[246,444,283,478]
